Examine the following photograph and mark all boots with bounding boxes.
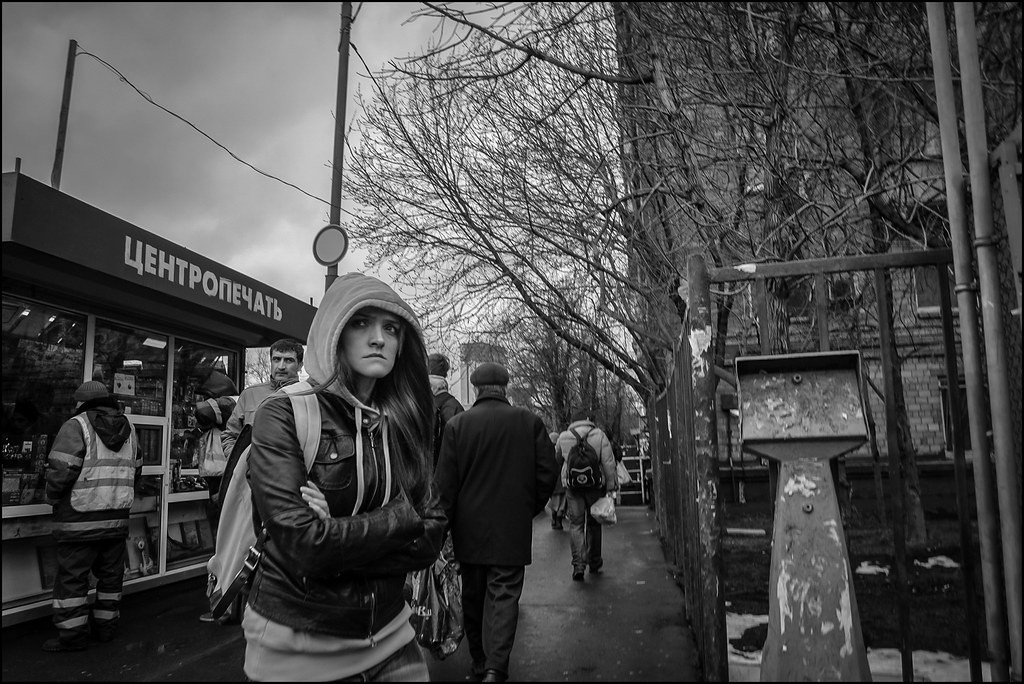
[551,510,558,529]
[556,515,563,531]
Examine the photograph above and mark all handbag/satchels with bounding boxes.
[616,460,633,487]
[591,492,617,524]
[411,564,464,659]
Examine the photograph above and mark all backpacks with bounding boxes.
[565,427,602,492]
[210,381,321,626]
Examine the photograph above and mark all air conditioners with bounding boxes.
[827,273,861,300]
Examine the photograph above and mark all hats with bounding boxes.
[470,362,511,386]
[73,381,109,401]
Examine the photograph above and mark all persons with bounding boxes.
[44,381,143,651]
[600,424,622,499]
[183,339,304,621]
[434,362,559,684]
[555,411,618,578]
[240,272,449,684]
[425,353,465,556]
[549,432,569,530]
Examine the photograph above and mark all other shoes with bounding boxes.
[200,609,233,622]
[573,565,585,579]
[40,637,86,651]
[588,556,602,573]
[482,669,500,683]
